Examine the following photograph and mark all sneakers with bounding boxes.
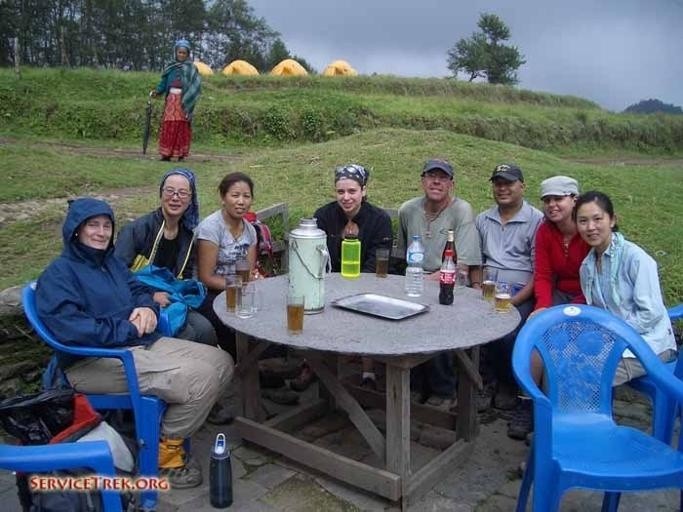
[208,403,233,426]
[158,454,203,489]
[260,365,315,404]
[413,391,458,411]
[508,405,533,440]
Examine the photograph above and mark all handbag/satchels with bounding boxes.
[133,264,208,337]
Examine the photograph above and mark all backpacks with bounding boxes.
[15,395,140,512]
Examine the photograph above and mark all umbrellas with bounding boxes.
[143,91,153,154]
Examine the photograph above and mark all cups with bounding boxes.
[494,283,512,314]
[375,248,390,278]
[481,268,498,300]
[225,259,305,338]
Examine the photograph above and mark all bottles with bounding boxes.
[441,231,457,267]
[404,236,425,297]
[341,234,361,278]
[208,432,233,508]
[438,249,456,304]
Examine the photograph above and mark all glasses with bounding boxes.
[161,187,194,199]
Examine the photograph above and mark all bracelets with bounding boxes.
[471,281,480,285]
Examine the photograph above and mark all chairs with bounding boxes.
[612,305,683,452]
[511,304,682,512]
[24,282,190,508]
[1,438,125,512]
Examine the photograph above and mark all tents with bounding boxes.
[324,60,358,77]
[269,59,308,77]
[223,59,260,76]
[190,62,214,75]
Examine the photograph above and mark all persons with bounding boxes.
[289,164,394,392]
[506,176,592,447]
[150,40,201,162]
[34,197,234,489]
[113,167,234,426]
[474,163,545,414]
[398,158,483,412]
[571,191,678,388]
[193,173,304,406]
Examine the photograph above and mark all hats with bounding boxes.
[423,158,454,176]
[540,175,580,200]
[489,164,524,183]
